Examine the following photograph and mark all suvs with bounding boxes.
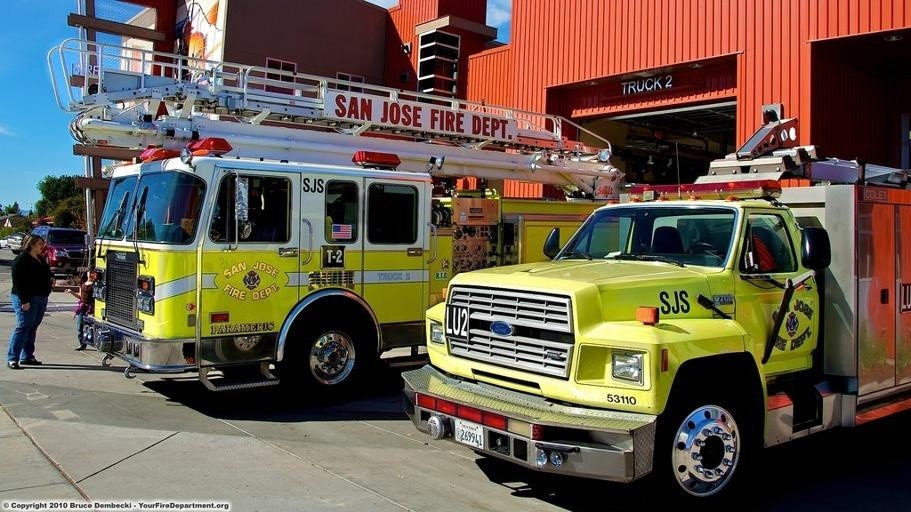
[31,226,92,281]
[8,232,27,254]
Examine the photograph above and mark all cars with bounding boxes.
[0,236,9,248]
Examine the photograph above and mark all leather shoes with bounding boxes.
[20,359,42,365]
[8,361,19,369]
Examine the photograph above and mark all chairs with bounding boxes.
[652,226,684,254]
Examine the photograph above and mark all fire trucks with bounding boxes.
[399,100,911,503]
[45,36,636,414]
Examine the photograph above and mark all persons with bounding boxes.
[7,235,56,369]
[64,265,94,351]
